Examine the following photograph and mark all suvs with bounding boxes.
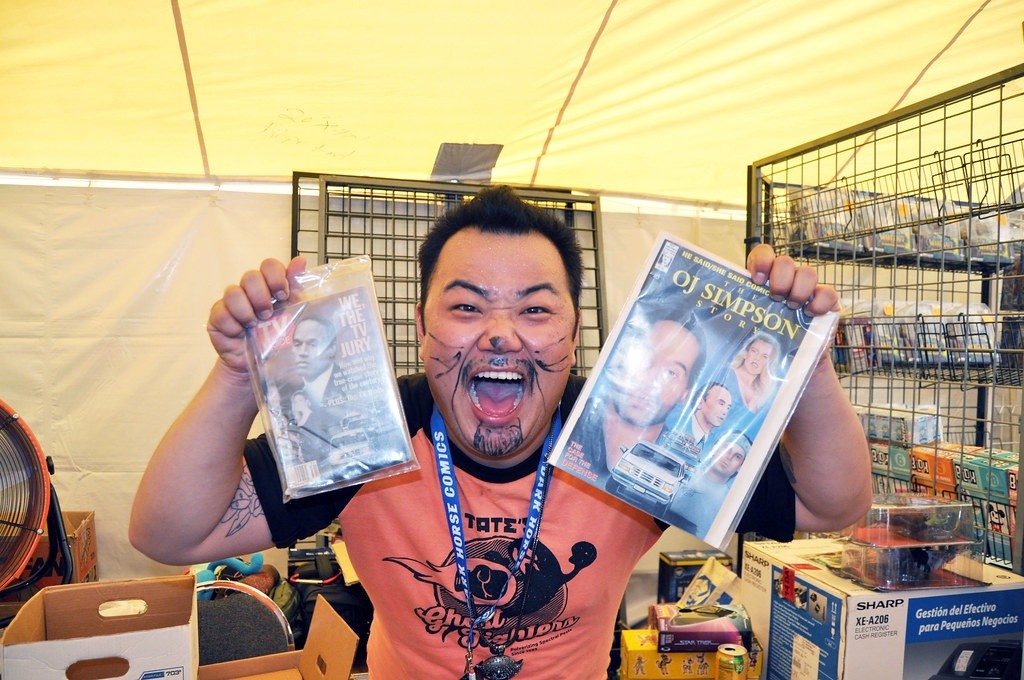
[327,411,382,473]
[605,441,689,519]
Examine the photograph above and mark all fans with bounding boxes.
[0,400,73,629]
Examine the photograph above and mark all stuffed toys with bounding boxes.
[194,552,264,602]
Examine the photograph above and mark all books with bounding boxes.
[241,253,416,499]
[543,227,841,554]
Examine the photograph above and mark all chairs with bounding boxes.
[197,580,295,666]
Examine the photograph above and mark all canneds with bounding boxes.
[716,643,749,680]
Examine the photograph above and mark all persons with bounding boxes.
[127,185,875,679]
[258,313,349,468]
[574,301,786,488]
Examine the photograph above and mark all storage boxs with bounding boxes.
[652,603,752,654]
[620,629,765,680]
[657,550,733,603]
[1008,464,1019,552]
[787,185,1024,375]
[858,401,944,493]
[739,538,1024,680]
[839,492,985,548]
[0,511,99,603]
[952,448,1019,561]
[908,440,985,501]
[0,574,200,680]
[826,542,993,593]
[198,594,360,680]
